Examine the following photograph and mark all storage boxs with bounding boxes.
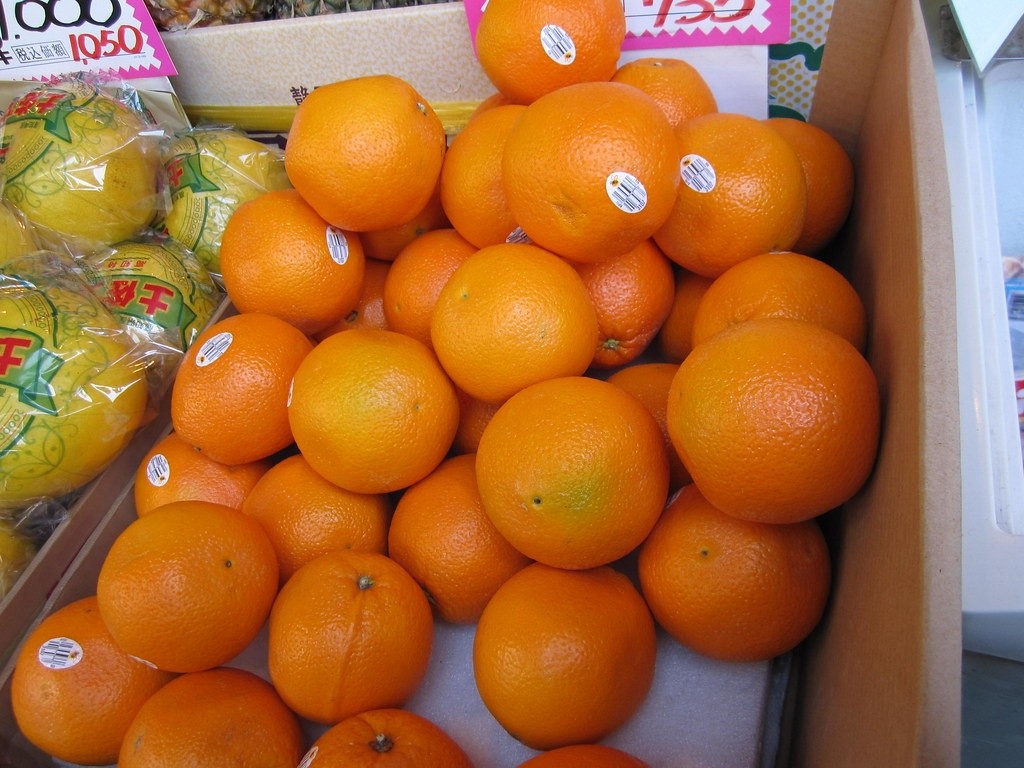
[2,0,966,768]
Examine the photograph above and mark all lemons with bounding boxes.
[0,89,293,606]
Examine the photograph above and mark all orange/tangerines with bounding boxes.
[12,0,880,768]
